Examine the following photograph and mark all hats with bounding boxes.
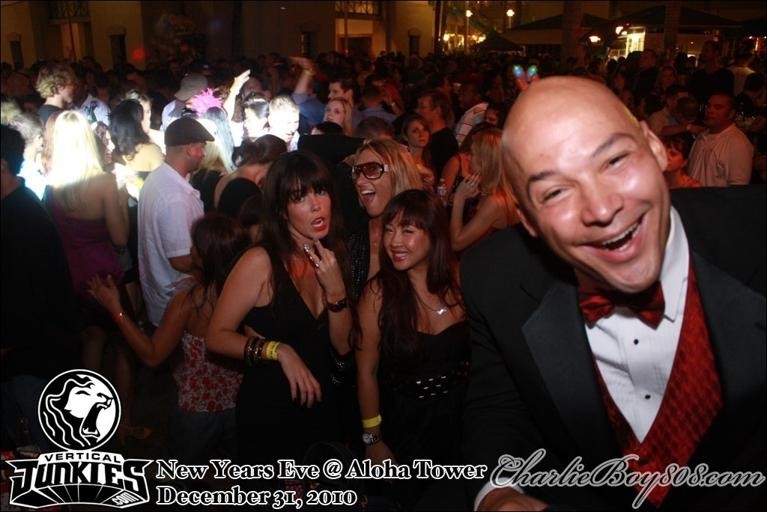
[163,117,214,146]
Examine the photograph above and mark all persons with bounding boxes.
[662,137,701,189]
[0,123,71,512]
[438,123,495,217]
[137,118,205,334]
[191,136,286,212]
[87,213,245,512]
[238,193,277,243]
[399,115,436,192]
[416,91,457,185]
[204,150,354,511]
[40,112,153,446]
[736,73,767,154]
[689,92,752,189]
[454,72,508,144]
[450,122,521,252]
[335,117,395,233]
[405,46,605,98]
[686,40,753,91]
[606,40,706,140]
[357,189,463,511]
[461,76,767,511]
[340,137,424,304]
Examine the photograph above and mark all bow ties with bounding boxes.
[576,279,666,330]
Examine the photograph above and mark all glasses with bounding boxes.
[350,161,386,181]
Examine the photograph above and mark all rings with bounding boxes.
[470,183,475,188]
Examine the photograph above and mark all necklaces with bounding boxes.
[420,296,448,315]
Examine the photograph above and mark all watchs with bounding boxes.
[363,432,382,444]
[325,294,349,312]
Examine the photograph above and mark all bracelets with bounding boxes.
[303,68,316,77]
[449,302,458,309]
[244,336,265,360]
[266,341,280,360]
[111,310,126,320]
[361,415,381,429]
